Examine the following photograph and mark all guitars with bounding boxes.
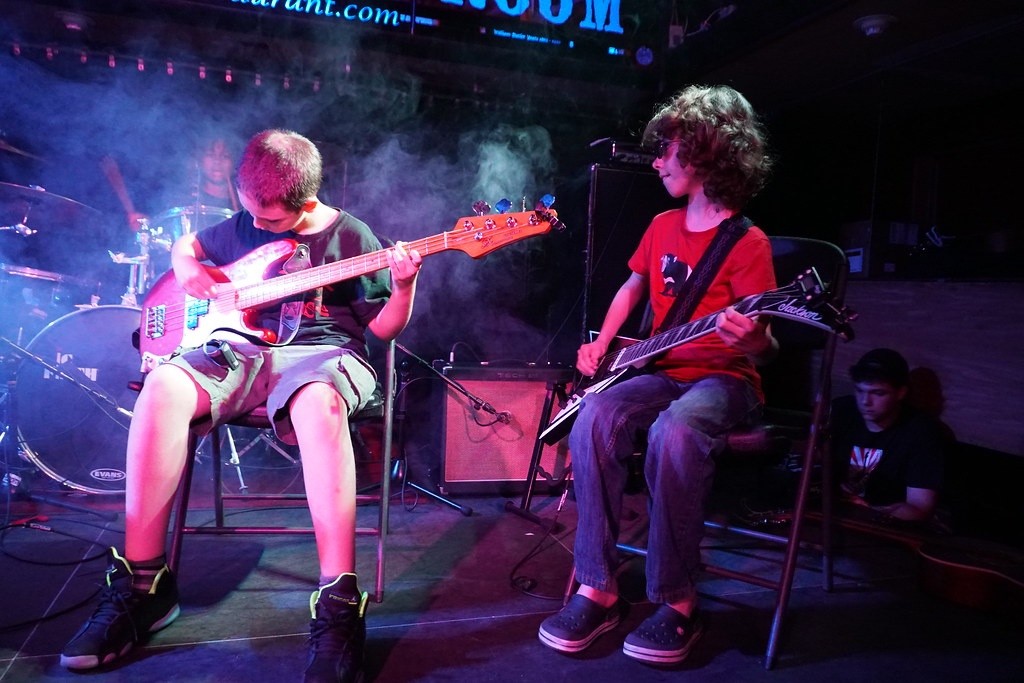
[747,491,1023,619]
[134,192,567,379]
[538,265,859,447]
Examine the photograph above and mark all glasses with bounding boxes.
[654,139,684,159]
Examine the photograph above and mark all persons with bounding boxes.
[59,128,424,682]
[537,84,783,663]
[127,129,244,233]
[806,346,958,540]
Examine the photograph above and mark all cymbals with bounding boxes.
[0,140,52,164]
[2,179,106,231]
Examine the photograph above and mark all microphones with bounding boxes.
[496,410,511,423]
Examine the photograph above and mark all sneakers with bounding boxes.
[61,546,181,669]
[303,573,368,683]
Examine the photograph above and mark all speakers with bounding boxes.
[441,365,591,497]
[582,164,689,345]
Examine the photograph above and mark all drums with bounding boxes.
[142,206,238,295]
[0,264,102,393]
[14,305,161,497]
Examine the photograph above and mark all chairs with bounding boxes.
[547,236,851,671]
[126,234,401,605]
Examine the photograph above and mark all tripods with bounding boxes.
[0,335,134,522]
[355,341,500,516]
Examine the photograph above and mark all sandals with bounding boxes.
[622,603,704,663]
[538,593,621,652]
